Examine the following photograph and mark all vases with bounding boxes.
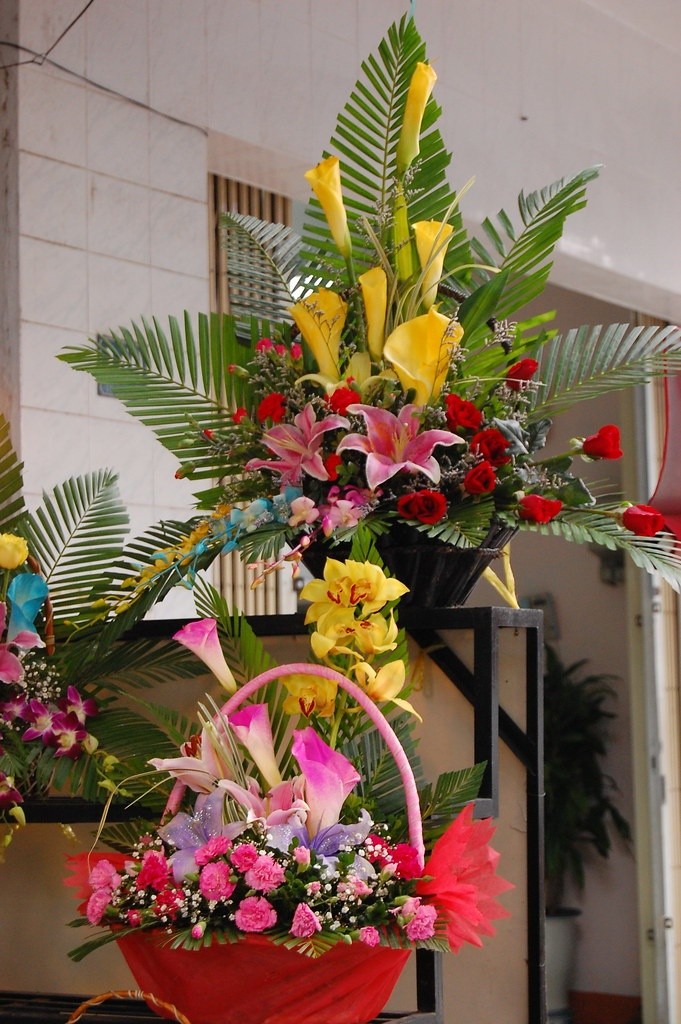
[287,527,521,610]
[111,923,411,1024]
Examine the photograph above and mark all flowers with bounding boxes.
[0,0,681,956]
